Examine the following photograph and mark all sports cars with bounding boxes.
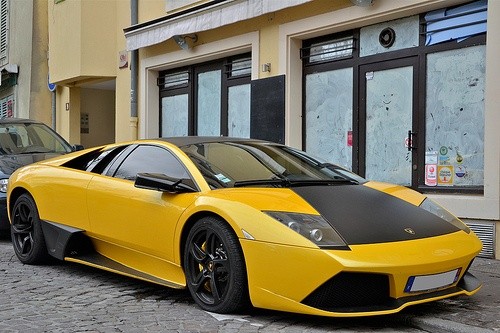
[7,135,482,318]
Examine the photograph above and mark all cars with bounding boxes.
[1,116,85,233]
